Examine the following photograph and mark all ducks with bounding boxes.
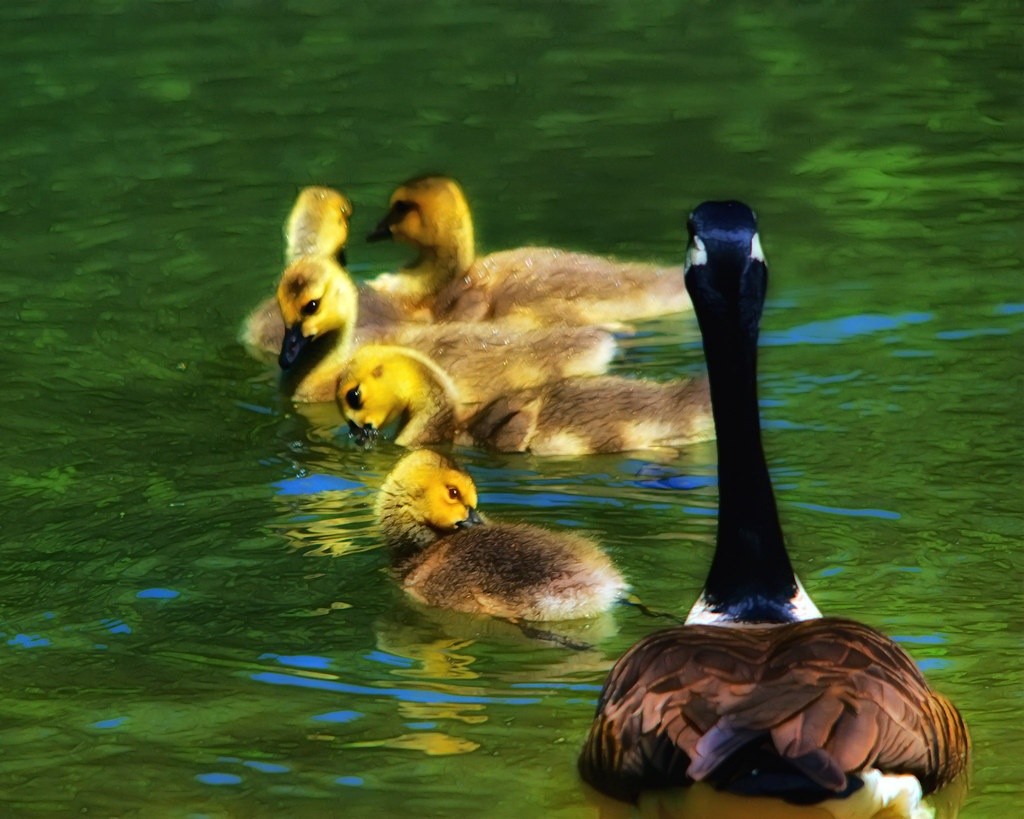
[336,346,716,453]
[376,450,683,651]
[578,196,978,819]
[368,174,693,327]
[237,186,616,406]
